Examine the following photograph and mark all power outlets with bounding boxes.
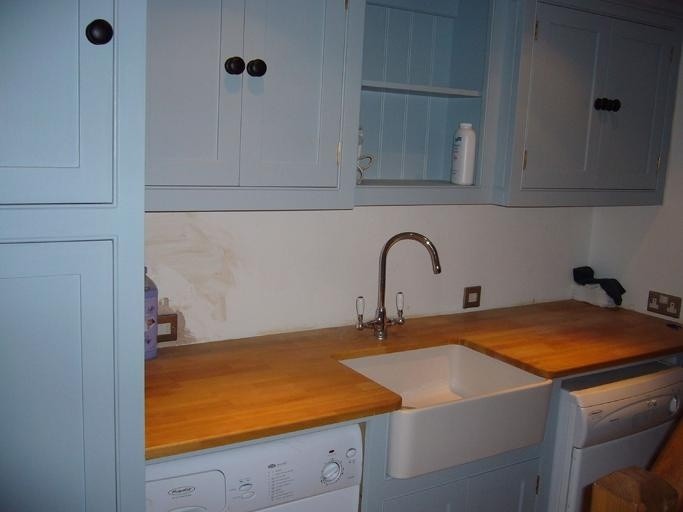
[647,289,682,320]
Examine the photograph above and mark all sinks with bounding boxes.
[338,345,552,477]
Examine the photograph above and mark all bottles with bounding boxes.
[144,266,174,360]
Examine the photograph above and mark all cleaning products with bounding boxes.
[144,266,158,360]
[451,122,477,184]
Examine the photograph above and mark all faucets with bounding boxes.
[378,232,441,308]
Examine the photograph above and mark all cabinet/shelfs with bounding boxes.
[0,0,144,511]
[144,0,366,217]
[360,446,553,512]
[356,3,495,208]
[500,0,683,211]
